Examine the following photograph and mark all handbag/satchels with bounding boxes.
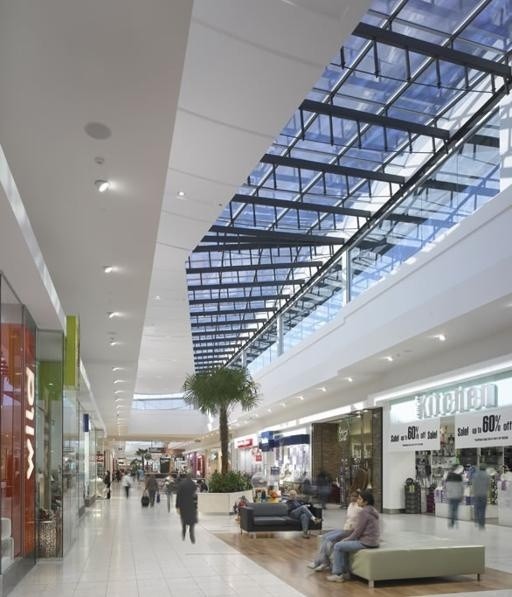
[156,493,160,503]
[193,494,198,509]
[127,482,131,488]
[142,496,149,506]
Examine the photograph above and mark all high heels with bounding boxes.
[306,560,330,572]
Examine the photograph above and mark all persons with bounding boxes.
[471,463,488,530]
[445,465,464,529]
[115,469,132,499]
[104,471,110,499]
[285,490,321,538]
[176,473,198,544]
[197,480,208,493]
[145,474,159,507]
[307,490,380,582]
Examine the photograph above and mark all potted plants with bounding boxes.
[183,364,264,515]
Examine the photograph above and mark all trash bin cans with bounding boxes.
[405,478,422,514]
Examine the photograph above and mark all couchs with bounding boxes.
[237,500,324,537]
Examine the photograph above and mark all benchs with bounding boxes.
[316,529,486,591]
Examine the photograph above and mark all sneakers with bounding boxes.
[303,533,310,539]
[326,572,351,582]
[314,518,321,525]
